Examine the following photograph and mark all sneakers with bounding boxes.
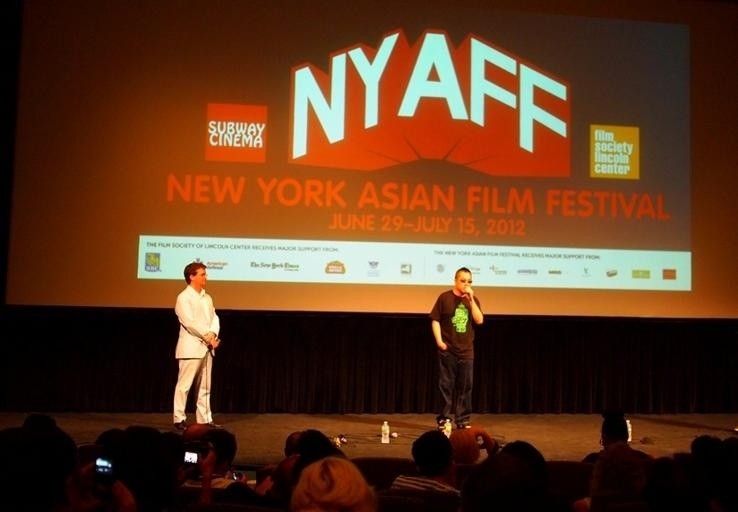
[437,421,471,431]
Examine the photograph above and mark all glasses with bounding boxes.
[460,281,473,283]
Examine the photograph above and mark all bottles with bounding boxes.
[443,419,453,440]
[381,421,390,444]
[625,419,632,442]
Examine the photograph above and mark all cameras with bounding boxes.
[95,455,112,474]
[184,451,198,465]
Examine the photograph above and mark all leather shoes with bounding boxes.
[206,422,225,429]
[174,421,187,431]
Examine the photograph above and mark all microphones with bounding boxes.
[464,285,473,304]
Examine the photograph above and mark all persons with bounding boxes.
[271,429,378,510]
[428,268,485,431]
[572,414,738,511]
[0,412,262,509]
[173,261,222,428]
[392,429,556,510]
